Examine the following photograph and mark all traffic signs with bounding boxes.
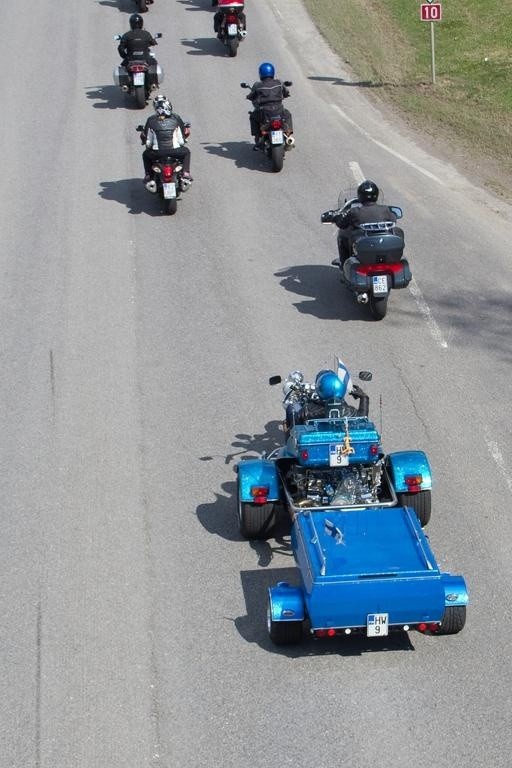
[421,4,441,21]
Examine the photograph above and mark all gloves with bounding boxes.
[349,385,365,399]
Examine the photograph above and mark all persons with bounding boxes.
[116,13,159,91]
[211,0,247,38]
[332,180,405,260]
[140,95,190,142]
[246,61,295,144]
[141,99,194,184]
[285,369,370,430]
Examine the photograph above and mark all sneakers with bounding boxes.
[182,172,194,182]
[143,174,150,183]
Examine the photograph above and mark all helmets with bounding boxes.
[314,369,345,400]
[257,63,275,79]
[357,180,379,203]
[153,94,172,111]
[129,13,144,29]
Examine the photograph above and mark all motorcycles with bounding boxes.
[217,0,247,56]
[240,81,295,172]
[114,33,163,109]
[136,125,191,215]
[321,188,412,319]
[136,0,153,12]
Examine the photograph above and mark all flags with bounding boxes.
[335,353,354,394]
